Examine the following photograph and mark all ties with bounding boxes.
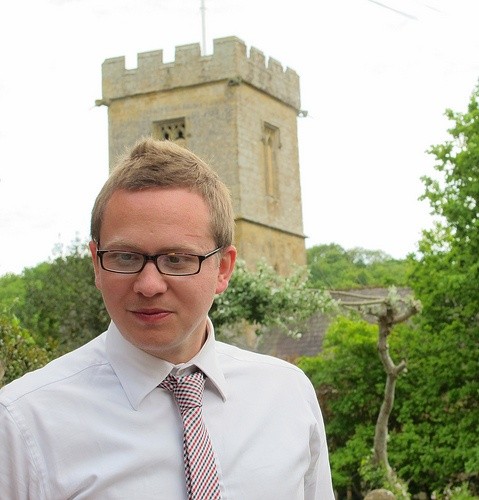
[157,369,222,500]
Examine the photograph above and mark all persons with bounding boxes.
[0,136,335,500]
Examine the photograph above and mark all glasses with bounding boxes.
[94,238,223,276]
